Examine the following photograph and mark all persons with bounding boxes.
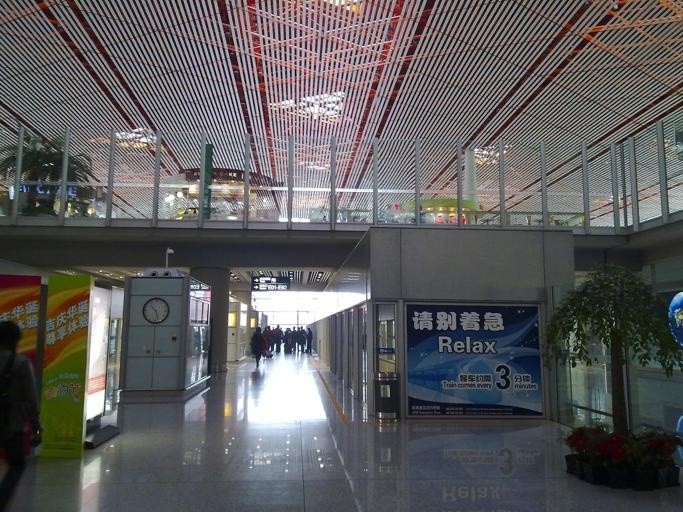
[250,323,314,368]
[0,319,45,512]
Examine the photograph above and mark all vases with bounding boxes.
[565,455,680,491]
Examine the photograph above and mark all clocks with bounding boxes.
[142,297,170,323]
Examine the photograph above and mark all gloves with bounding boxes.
[30,420,43,447]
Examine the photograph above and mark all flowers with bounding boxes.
[564,423,683,470]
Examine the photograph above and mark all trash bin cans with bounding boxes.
[375,372,401,419]
